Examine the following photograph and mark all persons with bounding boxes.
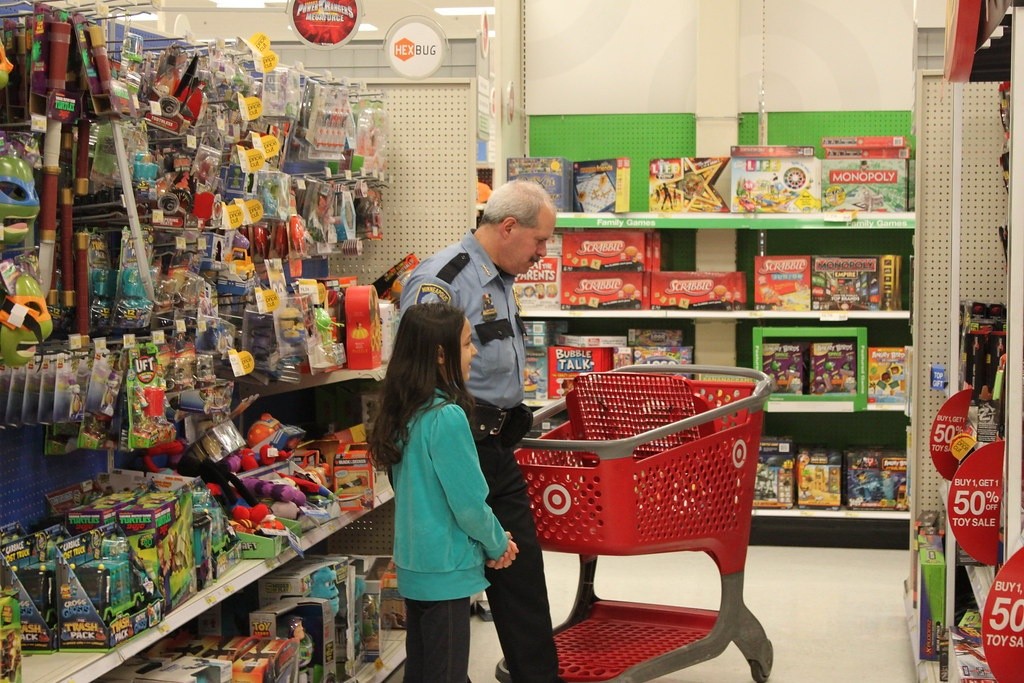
[367,303,520,683]
[400,179,559,683]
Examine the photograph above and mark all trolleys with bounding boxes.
[494,364,774,683]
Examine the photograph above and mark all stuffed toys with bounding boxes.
[145,438,328,526]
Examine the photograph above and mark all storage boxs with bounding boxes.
[0,441,405,683]
[958,610,982,627]
[948,627,997,683]
[505,135,916,514]
[918,534,947,661]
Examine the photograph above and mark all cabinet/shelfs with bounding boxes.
[521,212,914,520]
[0,362,407,683]
[909,472,998,683]
